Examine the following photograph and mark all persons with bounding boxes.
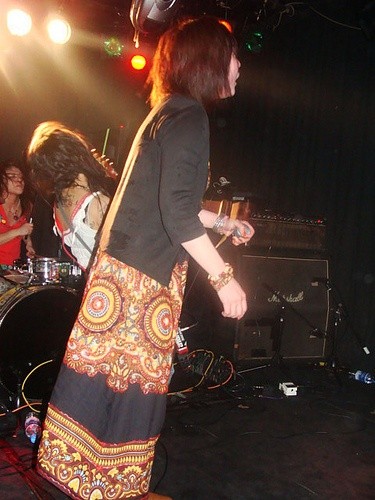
[0,163,35,266]
[28,120,117,272]
[36,15,255,500]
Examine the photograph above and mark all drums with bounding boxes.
[29,255,73,286]
[0,281,82,406]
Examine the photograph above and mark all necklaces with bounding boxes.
[9,209,18,220]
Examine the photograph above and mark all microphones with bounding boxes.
[312,331,327,337]
[312,277,329,284]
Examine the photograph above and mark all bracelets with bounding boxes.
[208,263,233,292]
[212,213,228,234]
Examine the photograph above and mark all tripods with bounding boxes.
[235,283,321,387]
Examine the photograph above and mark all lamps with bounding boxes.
[98,12,129,58]
[240,0,273,53]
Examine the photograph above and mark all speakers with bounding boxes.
[198,251,332,366]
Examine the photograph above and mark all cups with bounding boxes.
[35,258,70,281]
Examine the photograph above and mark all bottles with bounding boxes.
[25,412,42,444]
[347,369,375,384]
